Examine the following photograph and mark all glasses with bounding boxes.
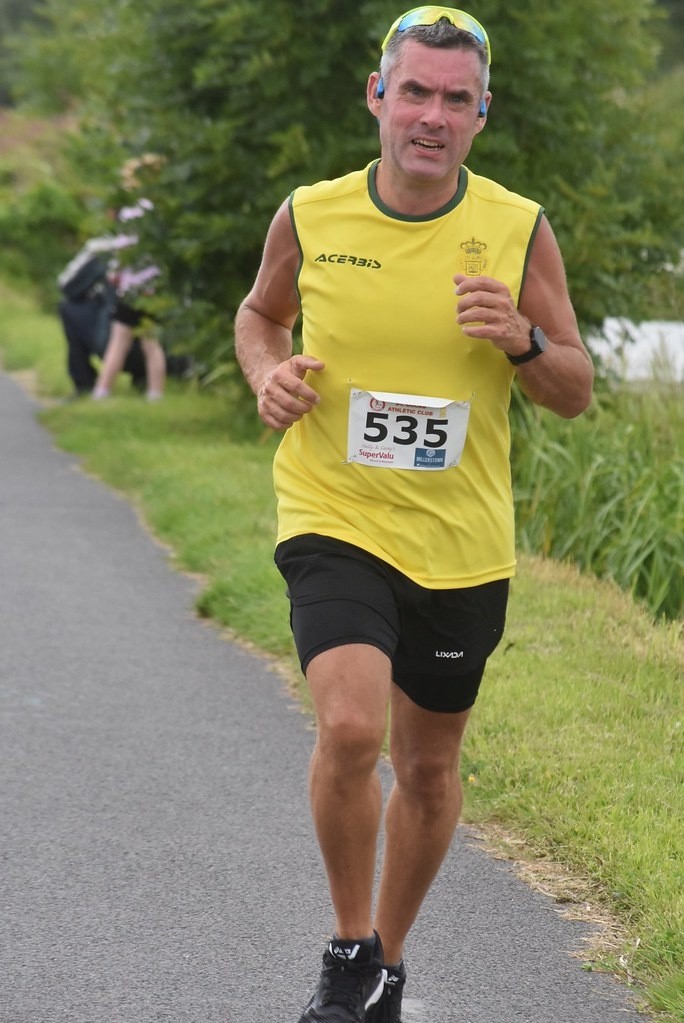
[381,7,493,68]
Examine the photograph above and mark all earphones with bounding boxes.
[477,100,486,119]
[376,78,385,99]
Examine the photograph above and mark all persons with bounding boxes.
[234,5,594,1022]
[91,157,167,397]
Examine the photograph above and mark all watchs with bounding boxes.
[504,323,548,365]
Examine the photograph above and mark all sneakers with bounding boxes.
[364,959,407,1023]
[296,929,386,1023]
